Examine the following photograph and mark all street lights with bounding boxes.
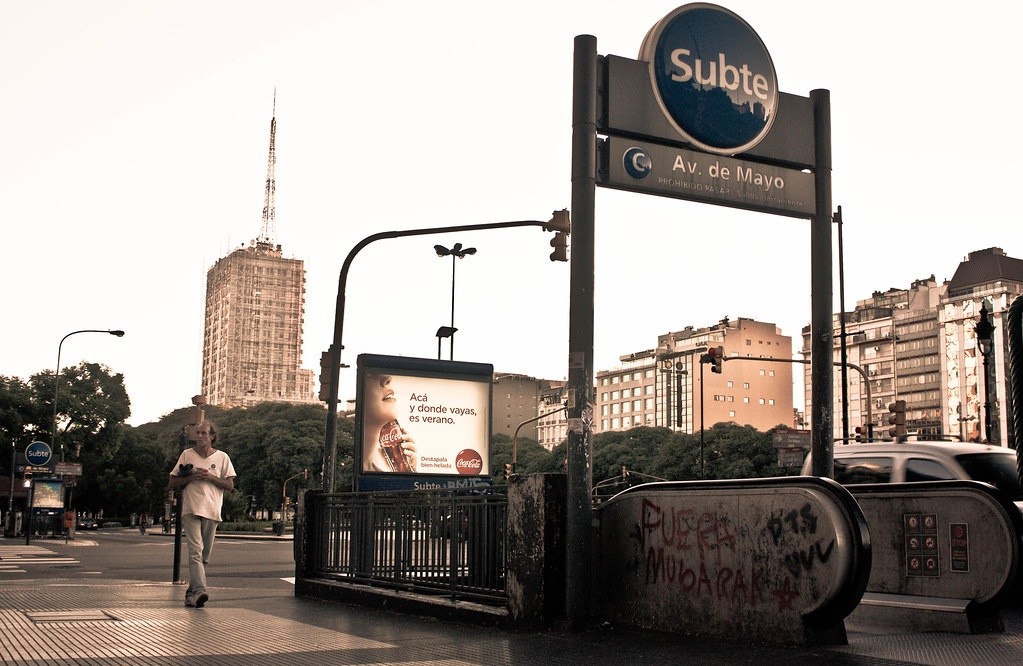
[435,327,459,360]
[48,329,125,454]
[433,241,477,360]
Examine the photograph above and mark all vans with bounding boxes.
[798,439,1023,524]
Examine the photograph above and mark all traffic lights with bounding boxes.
[305,468,313,482]
[855,426,869,444]
[707,346,724,376]
[285,496,291,506]
[281,497,285,503]
[888,399,910,439]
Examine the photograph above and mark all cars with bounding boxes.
[440,511,472,542]
[77,517,98,530]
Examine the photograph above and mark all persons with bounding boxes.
[140,515,146,534]
[167,420,237,608]
[363,373,417,472]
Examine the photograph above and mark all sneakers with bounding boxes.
[195,590,208,608]
[185,596,195,607]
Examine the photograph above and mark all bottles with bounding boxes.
[379,418,416,473]
